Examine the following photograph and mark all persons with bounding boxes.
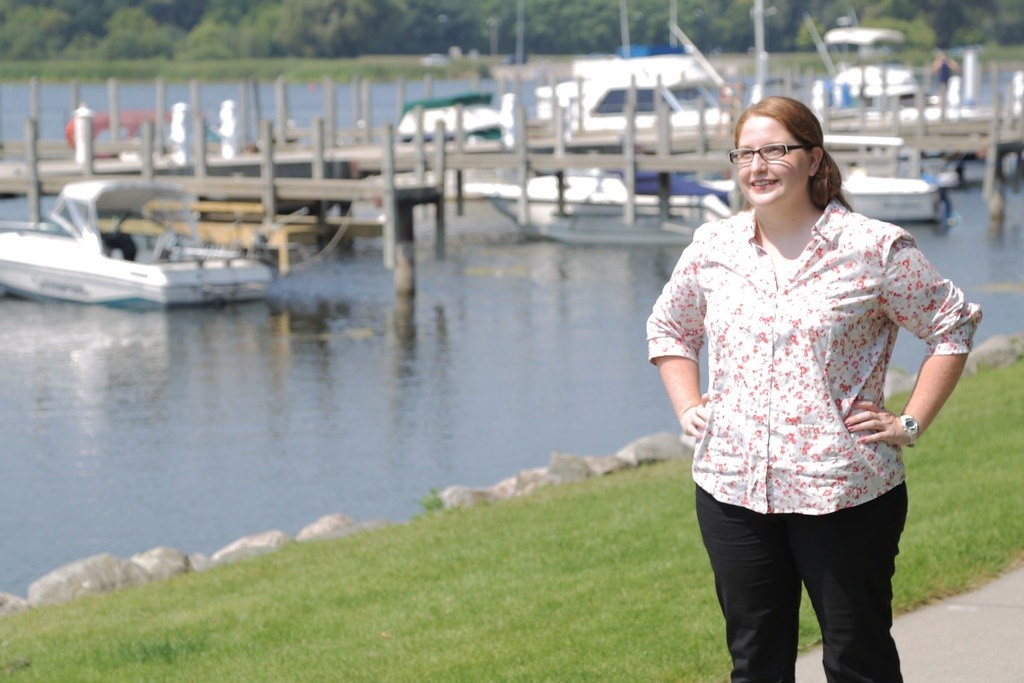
[646,96,983,683]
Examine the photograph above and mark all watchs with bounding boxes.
[899,415,919,447]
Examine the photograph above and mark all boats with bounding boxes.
[0,179,275,309]
[478,175,708,244]
[399,105,501,143]
[812,27,921,115]
[533,44,738,125]
[837,172,948,223]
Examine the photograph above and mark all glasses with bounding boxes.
[729,142,806,163]
